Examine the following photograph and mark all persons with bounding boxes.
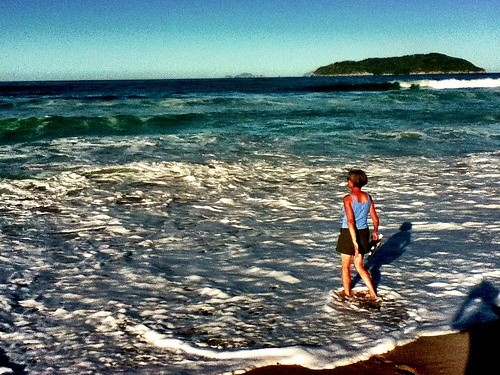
[334,169,380,301]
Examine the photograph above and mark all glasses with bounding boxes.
[346,176,351,181]
